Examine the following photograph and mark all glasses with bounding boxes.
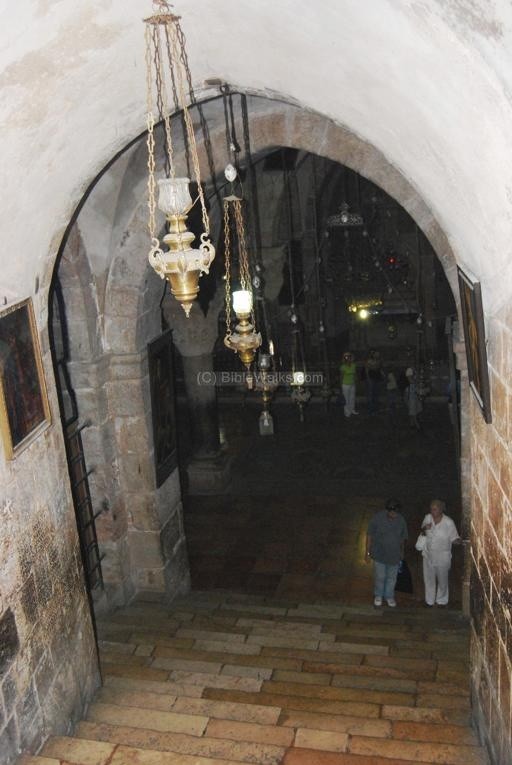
[388,503,403,511]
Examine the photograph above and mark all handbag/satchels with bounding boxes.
[415,534,427,551]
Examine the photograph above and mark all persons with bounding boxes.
[385,363,400,415]
[404,355,425,434]
[363,498,410,608]
[364,350,386,417]
[414,498,465,609]
[338,350,360,419]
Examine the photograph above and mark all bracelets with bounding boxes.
[365,552,372,556]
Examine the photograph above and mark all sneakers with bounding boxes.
[374,596,382,606]
[386,598,397,607]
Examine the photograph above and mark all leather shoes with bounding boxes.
[437,604,445,608]
[424,602,432,608]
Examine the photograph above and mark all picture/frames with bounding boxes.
[1,295,52,461]
[147,328,180,488]
[457,264,492,424]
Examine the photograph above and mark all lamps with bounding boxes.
[255,145,334,436]
[145,2,212,317]
[219,83,264,392]
